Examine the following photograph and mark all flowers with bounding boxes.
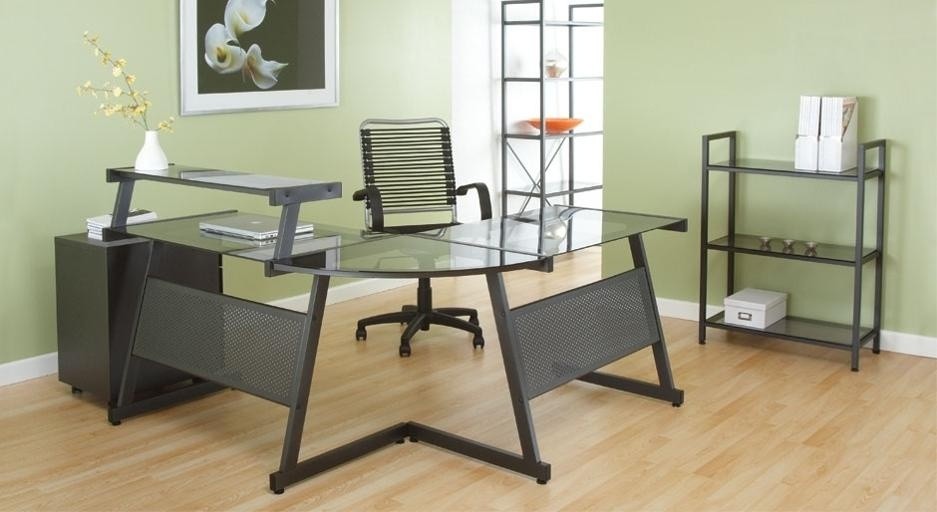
[75,30,176,137]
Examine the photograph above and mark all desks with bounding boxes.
[116,206,689,495]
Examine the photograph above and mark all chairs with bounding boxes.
[352,120,492,356]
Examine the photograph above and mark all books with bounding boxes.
[795,95,859,173]
[86,207,157,240]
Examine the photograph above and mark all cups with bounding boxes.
[805,242,819,249]
[759,244,770,251]
[805,249,817,256]
[760,237,771,243]
[781,240,794,246]
[782,247,793,254]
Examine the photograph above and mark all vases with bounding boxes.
[134,130,169,171]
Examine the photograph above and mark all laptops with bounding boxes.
[199,230,313,248]
[199,214,313,241]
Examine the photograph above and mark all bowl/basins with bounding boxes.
[525,118,582,134]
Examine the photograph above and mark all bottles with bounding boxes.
[543,43,568,78]
[541,214,567,253]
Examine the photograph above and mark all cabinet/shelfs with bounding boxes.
[700,132,887,373]
[54,232,224,426]
[500,2,602,215]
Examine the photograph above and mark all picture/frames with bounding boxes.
[179,1,340,116]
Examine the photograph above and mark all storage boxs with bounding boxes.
[722,287,787,331]
